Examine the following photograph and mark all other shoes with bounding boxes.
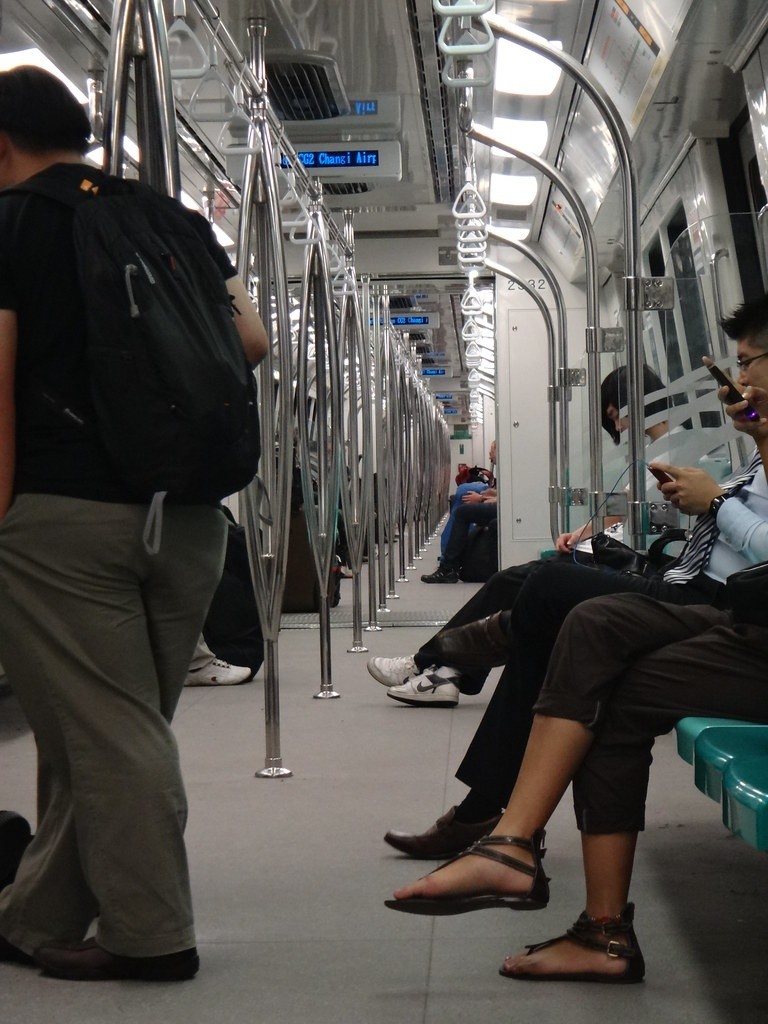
[362,547,389,562]
[340,565,353,578]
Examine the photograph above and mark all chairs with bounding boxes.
[672,717,768,853]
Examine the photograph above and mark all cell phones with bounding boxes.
[708,365,760,421]
[646,466,675,484]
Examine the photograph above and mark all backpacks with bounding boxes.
[459,517,498,583]
[203,525,269,681]
[0,179,261,554]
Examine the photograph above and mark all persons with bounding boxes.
[180,626,252,686]
[420,439,498,584]
[370,304,767,993]
[1,64,272,988]
[273,427,407,579]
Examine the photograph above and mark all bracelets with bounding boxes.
[708,492,733,519]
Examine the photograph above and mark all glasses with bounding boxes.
[737,352,768,368]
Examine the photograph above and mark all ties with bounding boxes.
[663,449,763,585]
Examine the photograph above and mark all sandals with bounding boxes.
[384,826,552,916]
[499,902,645,982]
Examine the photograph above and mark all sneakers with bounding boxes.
[367,654,459,707]
[183,659,252,686]
[421,565,458,584]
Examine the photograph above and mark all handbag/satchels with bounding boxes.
[591,528,692,580]
[726,561,768,627]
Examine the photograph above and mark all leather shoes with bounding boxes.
[384,805,505,860]
[434,609,508,671]
[38,936,200,981]
[0,933,35,965]
